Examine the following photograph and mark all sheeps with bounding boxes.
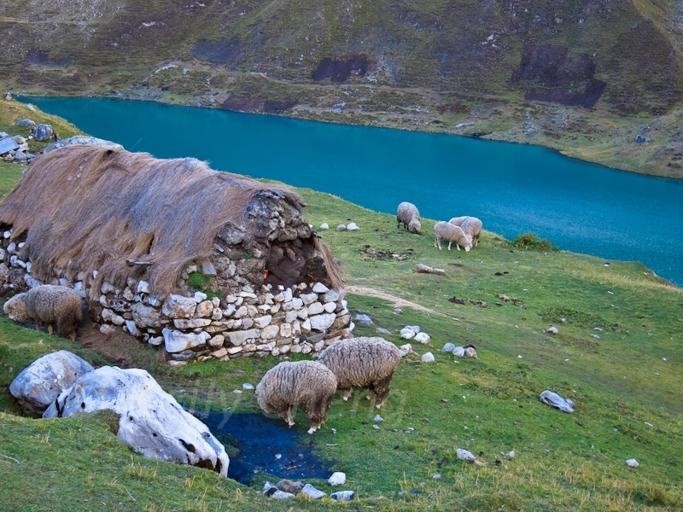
[3,284,83,343]
[460,217,483,247]
[448,216,469,227]
[315,336,401,409]
[396,201,422,234]
[433,220,473,252]
[254,359,338,435]
